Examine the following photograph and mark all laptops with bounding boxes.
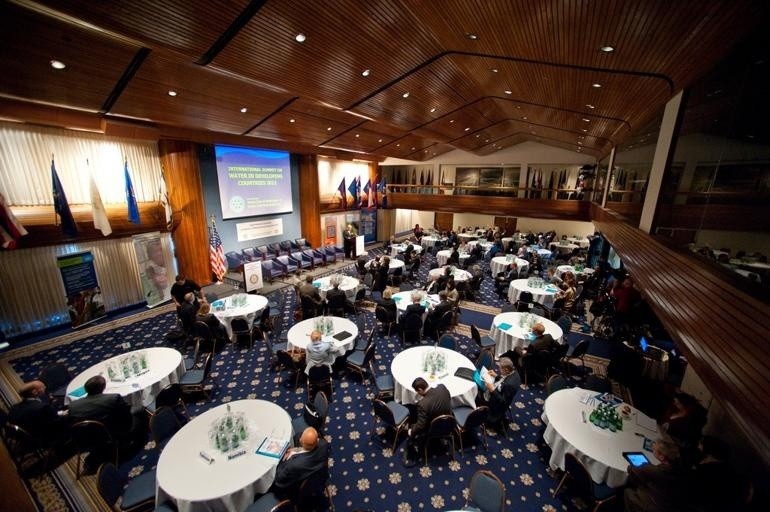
[638,336,648,352]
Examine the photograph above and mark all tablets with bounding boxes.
[622,450,652,467]
[643,437,655,451]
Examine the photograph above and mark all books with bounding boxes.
[68,382,88,398]
[256,435,289,457]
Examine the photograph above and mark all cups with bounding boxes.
[621,409,629,418]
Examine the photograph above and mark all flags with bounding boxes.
[160,169,174,232]
[529,169,580,199]
[124,163,141,225]
[0,194,28,250]
[52,159,80,236]
[338,171,444,211]
[612,171,637,202]
[87,169,113,237]
[210,223,226,282]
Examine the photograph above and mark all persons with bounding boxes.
[293,225,743,512]
[69,376,142,461]
[171,276,226,350]
[276,426,328,500]
[7,381,71,449]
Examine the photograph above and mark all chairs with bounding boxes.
[60,290,674,512]
[694,243,770,280]
[222,226,691,419]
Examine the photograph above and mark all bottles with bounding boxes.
[231,294,247,306]
[422,351,446,374]
[527,276,545,288]
[506,254,517,262]
[590,402,624,432]
[575,235,583,240]
[103,352,147,378]
[560,240,569,246]
[210,412,246,454]
[575,263,584,272]
[520,313,538,333]
[211,300,225,311]
[315,318,336,335]
